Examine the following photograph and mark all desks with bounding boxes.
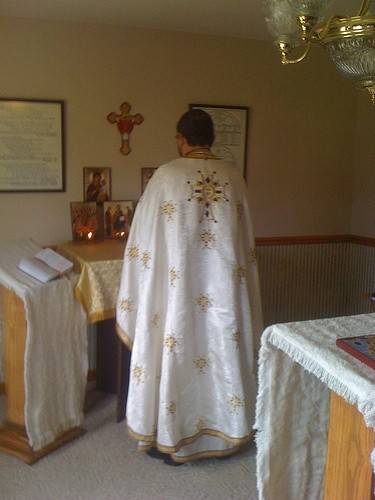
[250,312,375,500]
[57,239,129,424]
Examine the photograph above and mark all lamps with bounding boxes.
[262,0,375,107]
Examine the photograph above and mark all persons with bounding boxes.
[87,173,106,202]
[113,204,123,230]
[105,207,112,236]
[115,109,264,465]
[73,215,82,235]
[126,205,134,225]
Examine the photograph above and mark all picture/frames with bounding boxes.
[102,200,134,240]
[188,103,251,181]
[82,167,112,202]
[69,201,100,242]
[0,97,67,193]
[141,167,157,194]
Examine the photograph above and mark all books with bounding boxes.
[17,247,74,284]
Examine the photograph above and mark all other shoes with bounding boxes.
[146,447,184,466]
